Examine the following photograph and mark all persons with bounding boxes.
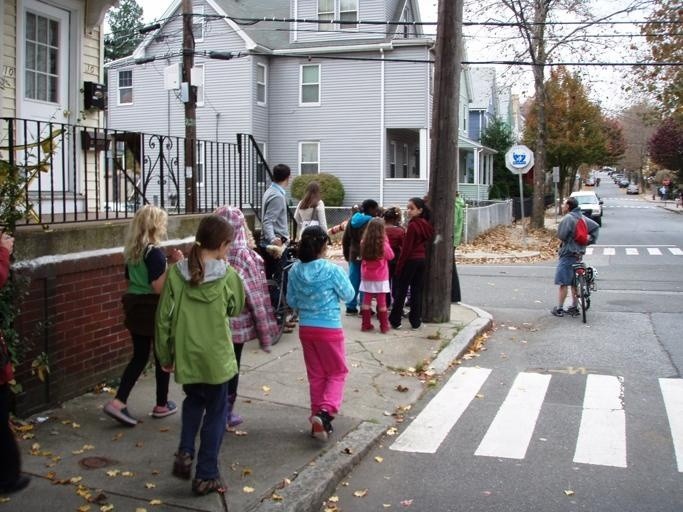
[155,216,246,495]
[342,199,378,316]
[361,218,390,334]
[0,231,29,494]
[285,226,355,442]
[261,163,295,333]
[450,188,465,303]
[102,206,184,426]
[550,198,600,318]
[327,203,362,235]
[294,182,327,245]
[386,207,404,249]
[390,198,433,330]
[213,203,278,427]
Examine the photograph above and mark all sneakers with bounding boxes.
[564,307,579,315]
[345,308,358,315]
[551,306,563,317]
[192,478,223,493]
[312,410,335,441]
[104,400,137,425]
[358,310,376,317]
[153,400,177,417]
[172,451,190,480]
[312,425,333,438]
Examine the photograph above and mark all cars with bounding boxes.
[626,184,639,195]
[618,178,630,188]
[568,191,602,229]
[585,174,596,185]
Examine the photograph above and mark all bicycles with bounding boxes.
[253,243,304,348]
[571,249,590,323]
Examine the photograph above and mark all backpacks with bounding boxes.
[568,213,588,245]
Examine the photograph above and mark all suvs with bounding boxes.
[614,174,626,184]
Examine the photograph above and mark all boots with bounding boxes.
[378,311,390,333]
[226,394,243,426]
[361,309,374,330]
